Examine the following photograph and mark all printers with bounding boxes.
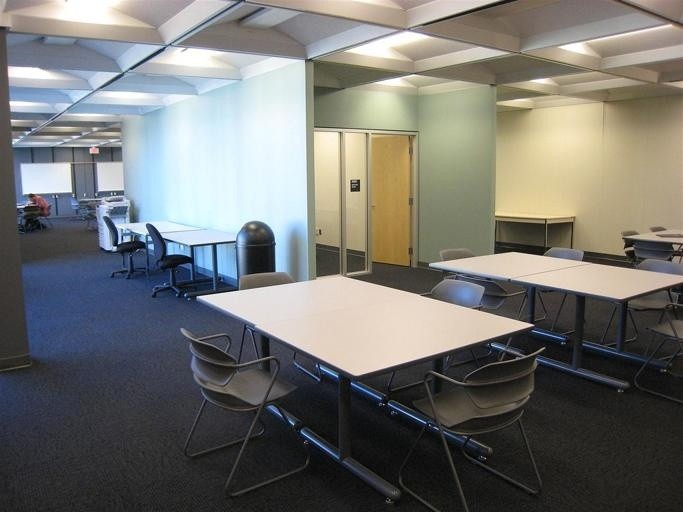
[95,194,132,253]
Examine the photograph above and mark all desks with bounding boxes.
[495,214,575,251]
[116,221,222,285]
[159,230,237,298]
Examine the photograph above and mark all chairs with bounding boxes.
[17,198,97,235]
[102,216,145,279]
[146,223,192,297]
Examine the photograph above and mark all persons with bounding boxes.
[24,192,50,232]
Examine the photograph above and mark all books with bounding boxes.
[656,233,683,238]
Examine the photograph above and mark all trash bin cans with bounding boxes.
[235,221,276,293]
